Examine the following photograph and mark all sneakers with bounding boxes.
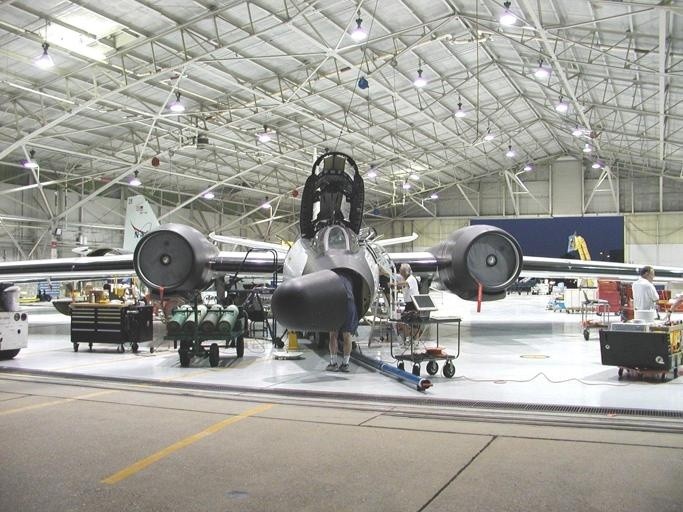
[326,363,349,372]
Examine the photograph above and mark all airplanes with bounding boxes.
[0,146,683,349]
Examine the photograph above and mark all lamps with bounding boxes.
[20,145,39,169]
[126,168,142,188]
[170,90,273,144]
[499,1,569,116]
[32,40,53,71]
[351,9,467,123]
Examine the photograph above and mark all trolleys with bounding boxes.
[579,289,610,341]
[598,327,683,384]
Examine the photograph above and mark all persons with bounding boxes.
[325,271,358,372]
[632,266,661,322]
[379,263,421,350]
